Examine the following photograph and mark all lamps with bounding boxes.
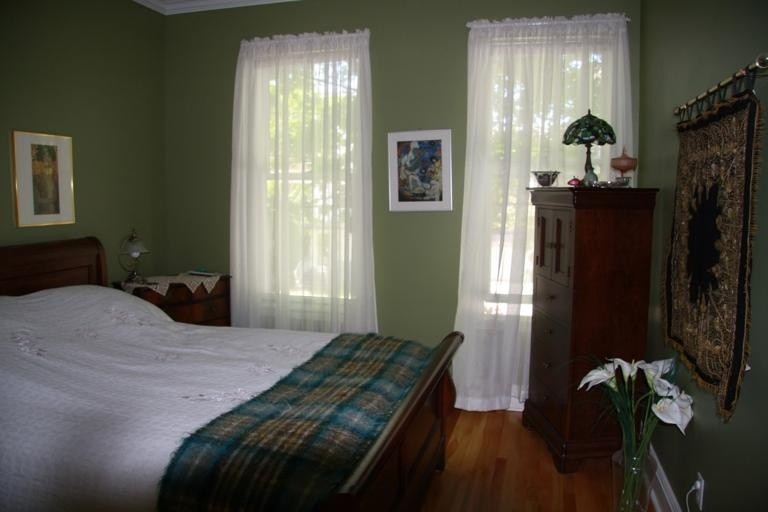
[563,107,615,186]
[117,228,149,284]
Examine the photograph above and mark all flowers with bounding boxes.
[552,351,693,511]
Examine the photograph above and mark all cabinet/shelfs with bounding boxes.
[522,188,661,471]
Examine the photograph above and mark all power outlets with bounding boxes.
[692,473,705,510]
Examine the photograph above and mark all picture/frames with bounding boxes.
[388,129,453,212]
[9,131,77,226]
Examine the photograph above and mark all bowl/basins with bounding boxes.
[532,171,560,187]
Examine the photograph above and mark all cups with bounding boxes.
[615,177,632,185]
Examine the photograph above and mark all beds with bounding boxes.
[0,236,464,512]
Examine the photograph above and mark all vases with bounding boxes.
[608,448,655,511]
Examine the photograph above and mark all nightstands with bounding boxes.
[111,273,232,328]
